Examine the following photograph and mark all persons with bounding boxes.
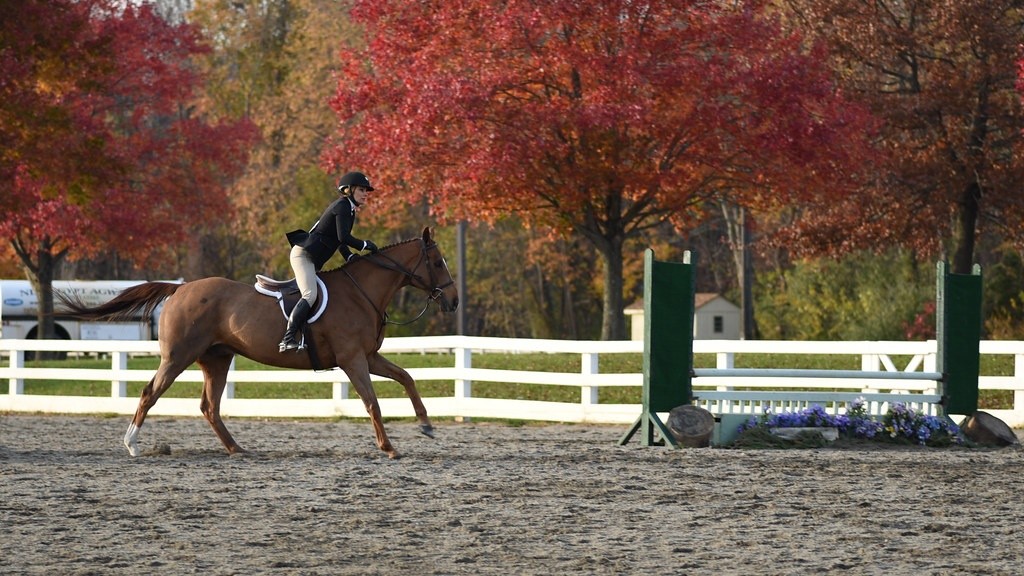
[278,171,376,353]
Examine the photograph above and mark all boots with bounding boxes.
[278,298,310,354]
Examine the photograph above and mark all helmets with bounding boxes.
[338,172,375,195]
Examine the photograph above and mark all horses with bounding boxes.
[25,226,459,461]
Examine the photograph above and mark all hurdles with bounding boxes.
[619,246,1018,451]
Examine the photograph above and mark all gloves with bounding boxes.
[364,240,377,250]
[350,253,360,260]
